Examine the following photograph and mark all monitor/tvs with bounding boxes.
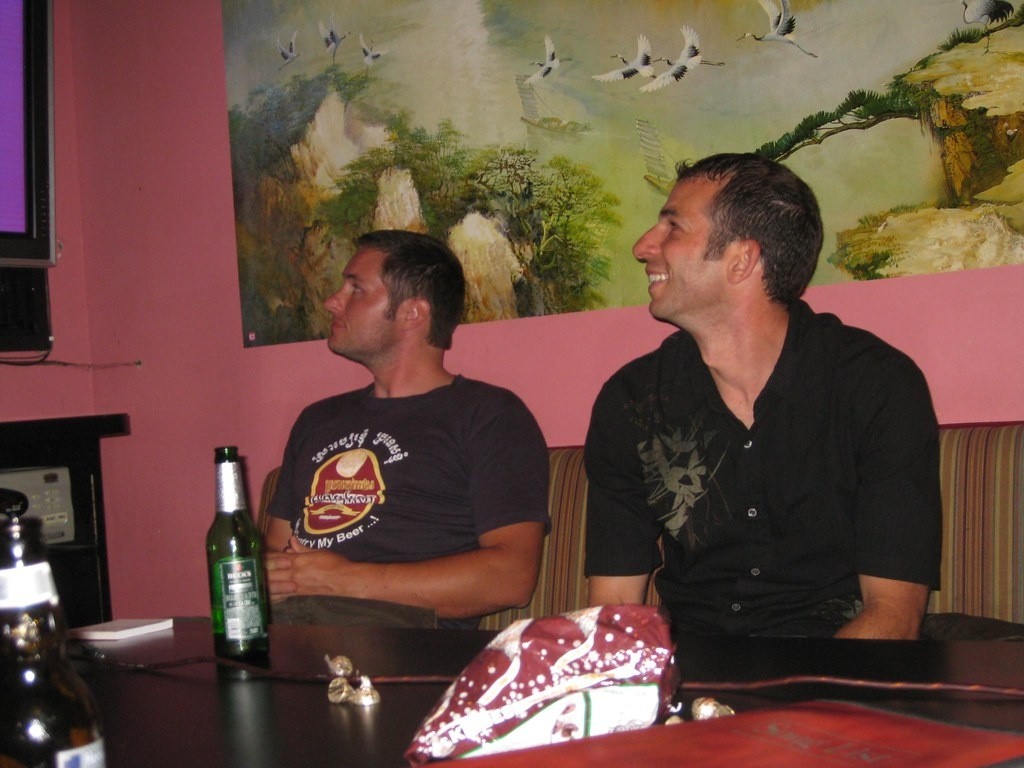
[0,0,56,267]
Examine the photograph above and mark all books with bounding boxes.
[65,617,174,641]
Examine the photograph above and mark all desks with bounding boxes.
[0,412,131,628]
[79,617,1024,768]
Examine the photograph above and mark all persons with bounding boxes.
[583,153,943,642]
[262,230,552,630]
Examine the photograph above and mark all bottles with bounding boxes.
[205,445,273,662]
[0,514,112,768]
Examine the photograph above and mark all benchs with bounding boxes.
[254,418,1024,629]
